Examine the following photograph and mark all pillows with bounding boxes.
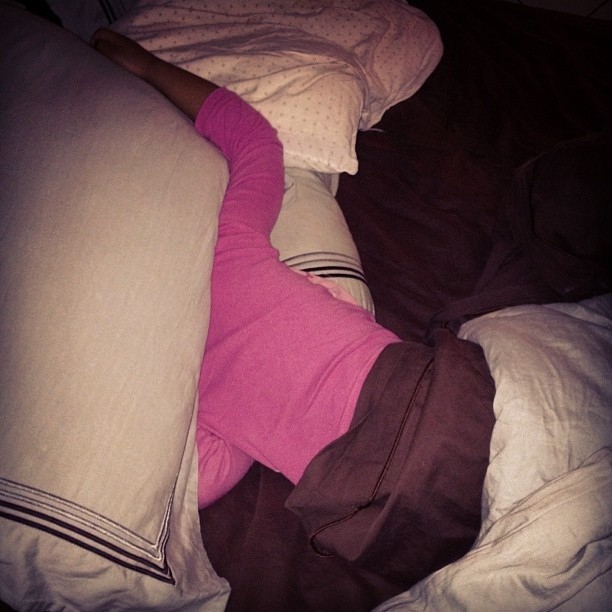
[1,24,231,612]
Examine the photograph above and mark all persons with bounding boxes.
[89,27,496,607]
